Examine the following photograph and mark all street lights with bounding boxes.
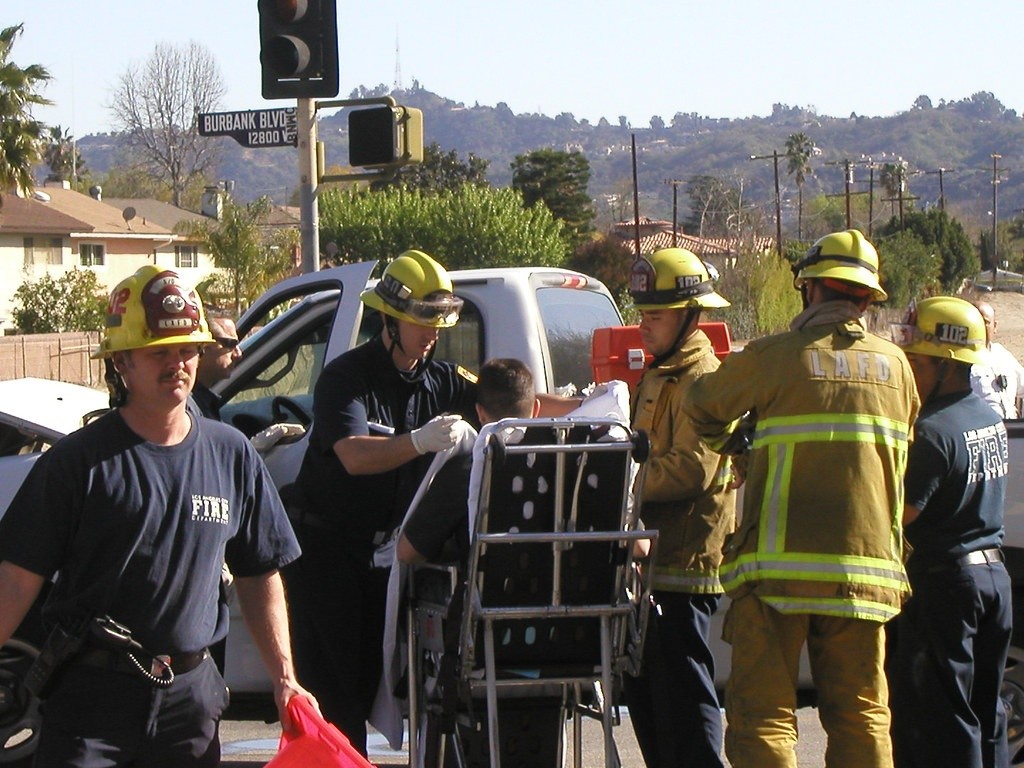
[751,145,956,262]
[979,151,1012,287]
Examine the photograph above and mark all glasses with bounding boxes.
[212,337,239,350]
[404,298,463,324]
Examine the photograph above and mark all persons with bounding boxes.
[629,247,737,768]
[278,250,584,761]
[0,265,325,768]
[679,229,923,768]
[193,316,306,677]
[890,296,1013,768]
[397,359,650,566]
[970,301,1024,419]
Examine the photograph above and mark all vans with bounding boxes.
[0,258,630,693]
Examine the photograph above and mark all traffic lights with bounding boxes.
[347,105,425,171]
[256,0,341,99]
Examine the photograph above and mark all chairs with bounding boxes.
[406,416,660,768]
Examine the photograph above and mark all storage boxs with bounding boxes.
[591,323,732,394]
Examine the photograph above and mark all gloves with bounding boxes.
[250,422,306,452]
[579,382,611,406]
[411,413,463,454]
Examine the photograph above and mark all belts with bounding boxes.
[77,646,206,680]
[943,545,1002,567]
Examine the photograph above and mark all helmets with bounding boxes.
[624,248,731,310]
[891,296,996,366]
[89,266,216,359]
[362,250,464,328]
[792,230,889,302]
